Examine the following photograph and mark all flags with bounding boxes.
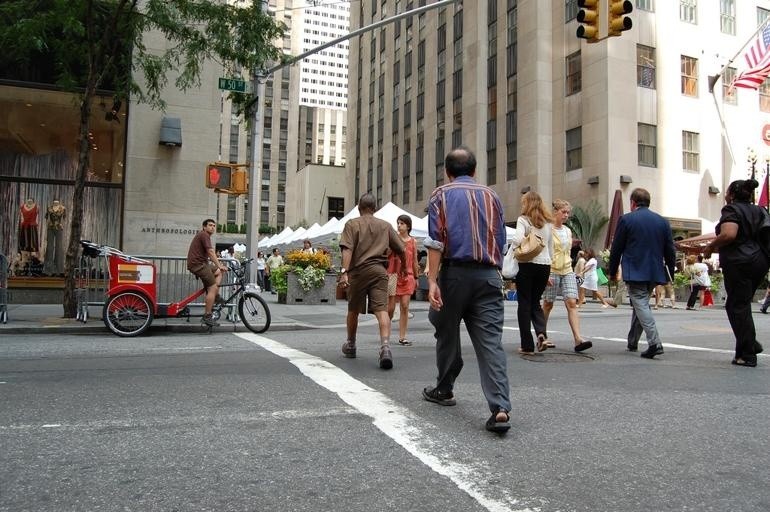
[757,173,770,208]
[727,24,770,96]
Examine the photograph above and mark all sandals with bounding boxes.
[485,409,514,432]
[422,385,458,406]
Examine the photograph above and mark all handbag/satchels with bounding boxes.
[512,232,546,262]
[757,207,770,248]
[388,272,399,298]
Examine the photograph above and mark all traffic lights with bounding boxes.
[608,0,632,37]
[205,163,235,190]
[577,0,599,43]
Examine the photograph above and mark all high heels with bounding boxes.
[517,346,535,356]
[536,331,548,352]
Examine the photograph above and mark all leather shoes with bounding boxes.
[624,344,639,353]
[639,342,665,360]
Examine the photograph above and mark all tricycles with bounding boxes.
[75,240,272,337]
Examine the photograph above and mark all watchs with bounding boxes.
[340,267,348,276]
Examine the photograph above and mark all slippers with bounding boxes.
[544,340,556,349]
[574,339,593,352]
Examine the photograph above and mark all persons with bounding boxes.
[226,245,238,290]
[543,198,593,353]
[300,239,319,255]
[16,197,41,270]
[571,187,714,358]
[511,191,556,356]
[43,200,67,278]
[703,179,770,366]
[422,146,511,431]
[216,249,222,257]
[187,218,228,327]
[759,286,770,314]
[257,247,285,294]
[337,192,409,368]
[386,214,422,347]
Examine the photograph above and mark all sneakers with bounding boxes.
[198,315,221,327]
[759,307,769,315]
[685,306,695,311]
[341,340,359,359]
[398,338,414,347]
[576,302,585,309]
[755,340,763,353]
[214,294,231,304]
[377,344,394,371]
[730,354,759,367]
[600,303,610,310]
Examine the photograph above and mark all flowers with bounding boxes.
[283,247,332,271]
